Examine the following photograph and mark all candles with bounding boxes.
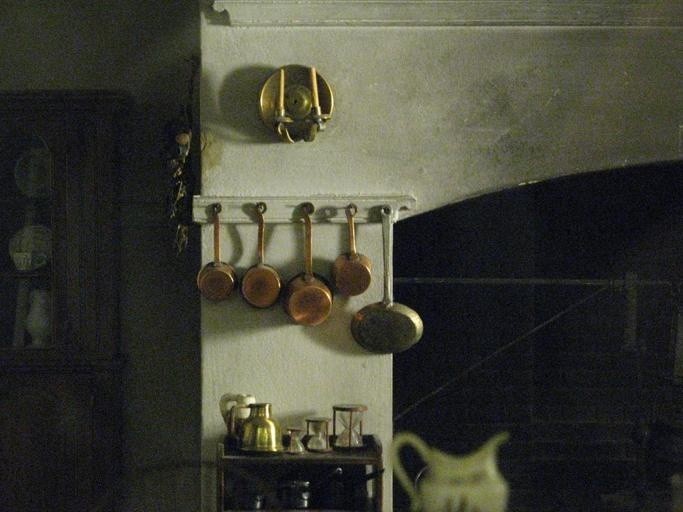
[275,68,285,117]
[310,67,322,116]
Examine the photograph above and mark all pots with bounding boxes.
[330,203,374,297]
[231,466,386,512]
[350,202,424,355]
[195,204,239,304]
[282,201,334,328]
[240,202,284,309]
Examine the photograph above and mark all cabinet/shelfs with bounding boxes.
[215,434,383,512]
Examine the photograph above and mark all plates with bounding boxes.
[7,223,55,273]
[14,146,52,198]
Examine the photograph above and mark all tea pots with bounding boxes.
[386,430,513,512]
